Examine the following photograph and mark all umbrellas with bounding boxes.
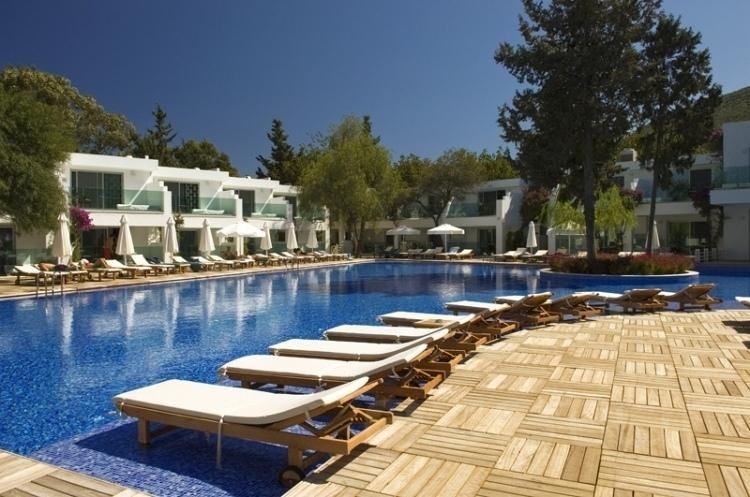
[50,210,72,265]
[199,219,215,258]
[426,224,465,253]
[286,221,299,252]
[385,224,420,247]
[526,220,538,253]
[115,215,135,265]
[306,223,319,252]
[260,222,273,255]
[217,215,266,256]
[644,220,660,253]
[164,217,179,257]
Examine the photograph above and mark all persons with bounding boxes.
[103,234,115,259]
[331,242,336,253]
[84,258,111,269]
[39,261,72,271]
[223,246,236,259]
[356,241,363,258]
[294,248,307,256]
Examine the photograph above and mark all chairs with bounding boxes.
[373,246,474,259]
[11,255,252,286]
[735,296,749,308]
[247,251,348,267]
[491,248,549,261]
